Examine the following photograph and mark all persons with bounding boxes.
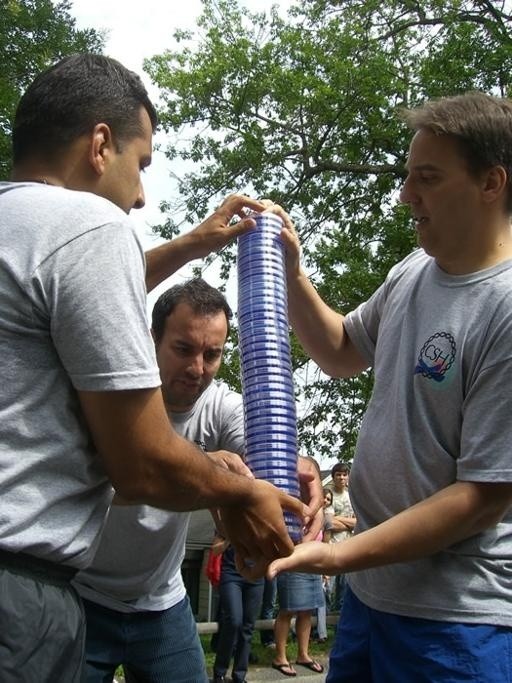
[227,88,511,683]
[71,278,330,683]
[208,454,358,683]
[0,52,310,683]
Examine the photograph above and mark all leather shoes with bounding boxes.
[212,671,225,683]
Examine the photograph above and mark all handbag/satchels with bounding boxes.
[203,532,225,587]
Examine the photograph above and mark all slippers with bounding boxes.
[296,657,325,674]
[270,658,296,677]
